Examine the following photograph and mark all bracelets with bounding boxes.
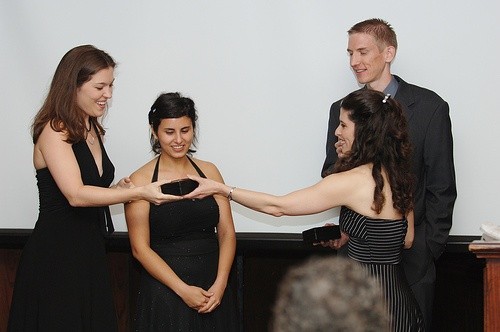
[227,187,233,201]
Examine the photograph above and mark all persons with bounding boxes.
[270,255,390,332]
[125,93,236,332]
[7,45,184,332]
[183,89,414,332]
[321,19,457,332]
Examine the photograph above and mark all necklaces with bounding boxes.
[87,129,96,145]
